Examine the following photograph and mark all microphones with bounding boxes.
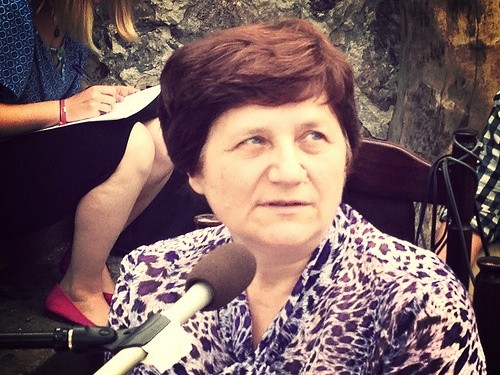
[93,243,257,375]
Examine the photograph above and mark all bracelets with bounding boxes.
[60,100,66,125]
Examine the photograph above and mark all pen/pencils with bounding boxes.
[70,65,96,87]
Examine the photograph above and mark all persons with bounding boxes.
[0,0,174,326]
[433,92,500,271]
[104,18,487,375]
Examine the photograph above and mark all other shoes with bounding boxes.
[44,283,97,327]
[59,250,114,306]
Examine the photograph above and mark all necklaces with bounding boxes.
[54,24,60,38]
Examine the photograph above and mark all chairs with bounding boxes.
[340,126,479,291]
